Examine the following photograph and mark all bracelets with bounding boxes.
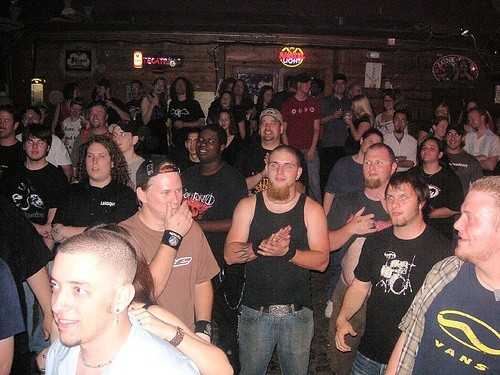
[194,321,212,336]
[281,245,296,261]
[252,239,264,257]
[395,156,399,163]
[161,230,183,251]
[168,326,185,347]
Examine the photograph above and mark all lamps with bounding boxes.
[460,28,469,35]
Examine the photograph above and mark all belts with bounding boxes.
[245,299,302,317]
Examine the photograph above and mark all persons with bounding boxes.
[0,73,500,375]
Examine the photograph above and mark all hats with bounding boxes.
[136,155,178,187]
[259,108,283,125]
[109,120,138,136]
[446,123,464,136]
[333,73,347,84]
[295,73,312,83]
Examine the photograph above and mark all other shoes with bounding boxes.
[325,301,333,318]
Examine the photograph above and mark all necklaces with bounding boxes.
[79,349,112,368]
